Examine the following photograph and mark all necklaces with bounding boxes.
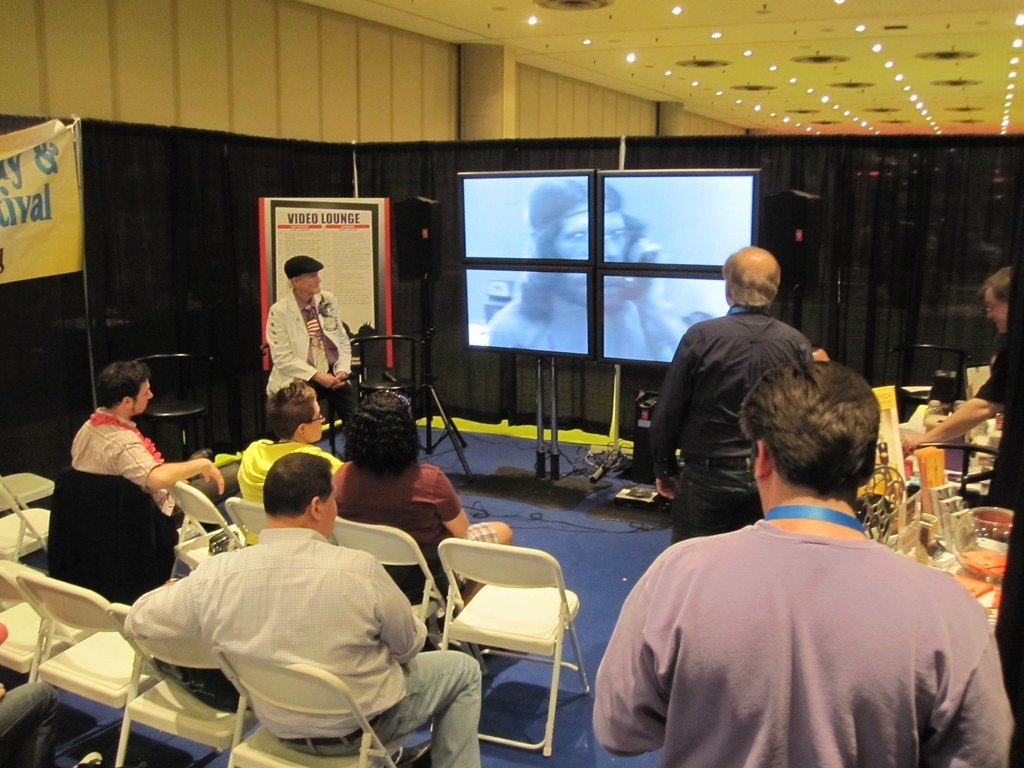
[90,412,164,463]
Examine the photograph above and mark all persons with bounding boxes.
[488,178,687,362]
[902,266,1015,512]
[237,380,345,546]
[123,452,482,768]
[71,359,242,530]
[0,622,104,768]
[332,390,513,659]
[266,256,360,426]
[650,246,815,546]
[592,361,1015,768]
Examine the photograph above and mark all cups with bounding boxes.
[900,510,947,566]
[952,506,1013,579]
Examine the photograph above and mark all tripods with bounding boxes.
[410,282,477,484]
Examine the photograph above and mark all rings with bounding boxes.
[908,447,914,453]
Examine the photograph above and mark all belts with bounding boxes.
[685,452,753,469]
[285,714,383,744]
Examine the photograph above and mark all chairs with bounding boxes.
[890,343,972,420]
[0,333,592,768]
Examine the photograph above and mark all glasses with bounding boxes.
[986,300,1007,312]
[307,415,325,424]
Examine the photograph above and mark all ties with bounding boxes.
[306,304,329,376]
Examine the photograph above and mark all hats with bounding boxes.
[284,255,324,280]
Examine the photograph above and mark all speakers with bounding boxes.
[762,190,826,301]
[395,196,442,282]
[632,391,662,483]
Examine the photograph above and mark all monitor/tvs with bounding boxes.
[458,167,761,367]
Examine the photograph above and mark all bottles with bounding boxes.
[923,399,942,433]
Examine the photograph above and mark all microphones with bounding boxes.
[340,372,359,381]
